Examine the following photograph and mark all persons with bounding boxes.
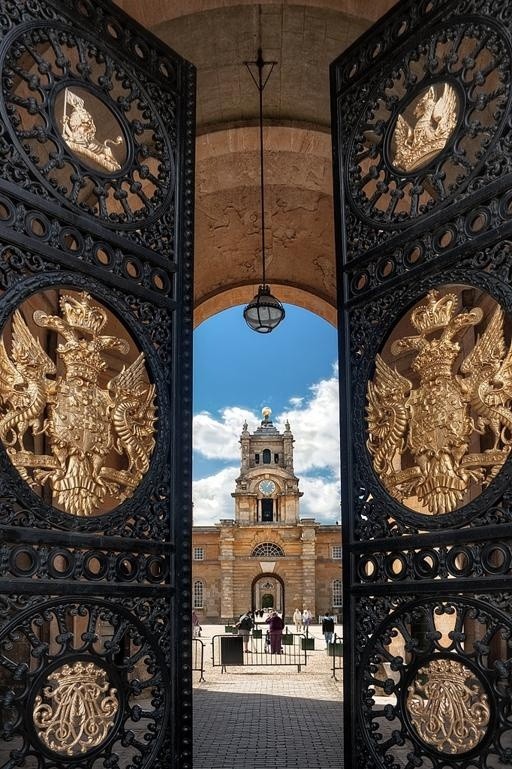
[192,610,200,639]
[238,610,255,653]
[294,608,302,632]
[303,609,308,633]
[266,613,285,655]
[322,612,334,648]
[306,608,312,625]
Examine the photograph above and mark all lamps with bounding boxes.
[242,1,287,333]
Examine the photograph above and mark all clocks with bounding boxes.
[258,480,277,495]
